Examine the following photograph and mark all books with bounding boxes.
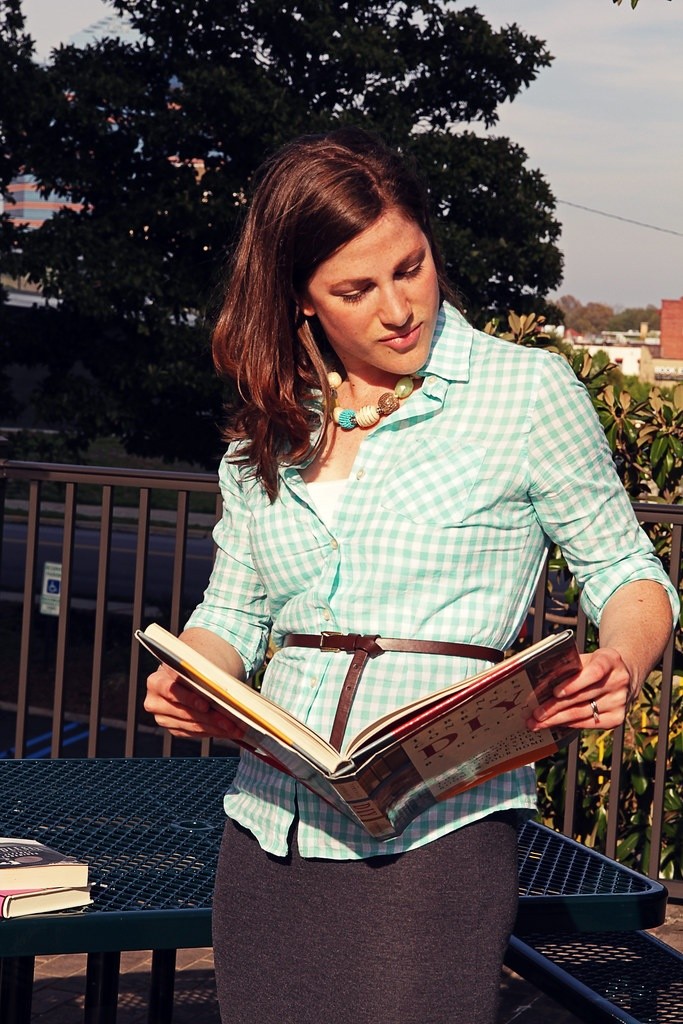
[0,837,95,918]
[135,620,589,843]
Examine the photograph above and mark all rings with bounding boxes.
[590,699,600,720]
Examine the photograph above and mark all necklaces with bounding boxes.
[321,364,423,428]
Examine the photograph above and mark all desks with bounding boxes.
[0,756,667,1023]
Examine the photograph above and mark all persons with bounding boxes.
[143,129,681,1023]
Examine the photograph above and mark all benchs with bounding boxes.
[499,931,682,1024]
